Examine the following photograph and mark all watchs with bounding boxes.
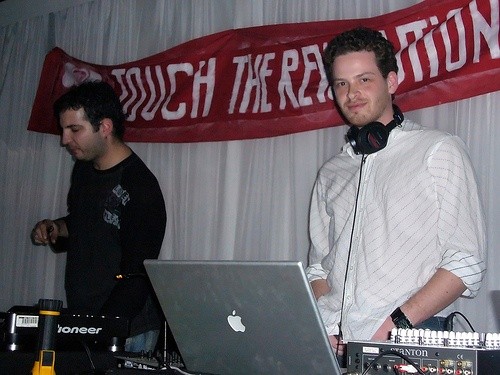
[390,307,414,332]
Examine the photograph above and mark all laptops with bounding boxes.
[143,259,344,375]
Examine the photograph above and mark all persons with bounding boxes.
[30,80,167,354]
[304,26,489,371]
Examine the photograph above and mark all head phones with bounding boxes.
[344,104,403,156]
